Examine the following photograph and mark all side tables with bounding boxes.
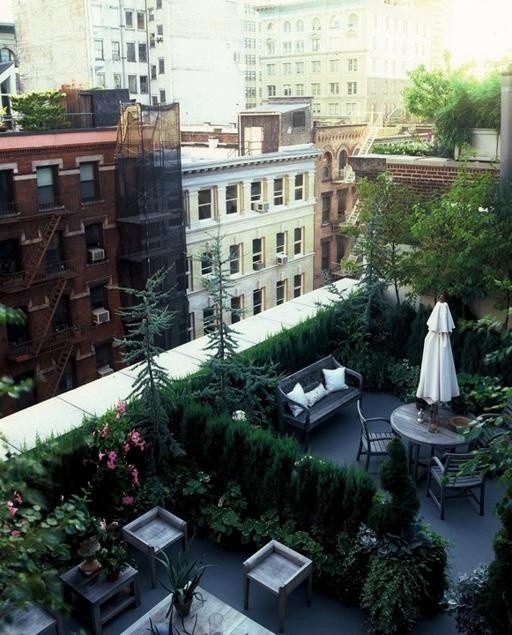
[58,560,140,635]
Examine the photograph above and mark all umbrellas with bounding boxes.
[416,293,462,431]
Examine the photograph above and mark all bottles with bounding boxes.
[417,408,425,423]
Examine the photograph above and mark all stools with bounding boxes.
[122,507,187,589]
[0,604,58,635]
[243,540,313,633]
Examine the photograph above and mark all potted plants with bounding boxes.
[95,546,128,582]
[208,137,218,149]
[152,545,218,617]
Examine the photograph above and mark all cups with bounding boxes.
[428,415,439,433]
[207,613,223,633]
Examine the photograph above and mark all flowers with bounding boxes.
[78,537,100,557]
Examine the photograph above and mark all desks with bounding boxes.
[390,403,482,488]
[119,582,276,635]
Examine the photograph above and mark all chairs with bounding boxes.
[426,455,485,520]
[478,405,512,480]
[356,400,401,472]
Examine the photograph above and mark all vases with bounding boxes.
[79,558,102,576]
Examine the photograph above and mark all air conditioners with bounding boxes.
[88,249,104,261]
[256,203,269,211]
[98,365,114,377]
[91,308,109,325]
[277,256,287,265]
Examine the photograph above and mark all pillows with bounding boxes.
[305,384,328,408]
[286,383,307,418]
[322,368,349,393]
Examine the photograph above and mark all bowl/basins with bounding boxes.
[448,416,472,432]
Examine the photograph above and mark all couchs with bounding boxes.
[276,355,363,453]
